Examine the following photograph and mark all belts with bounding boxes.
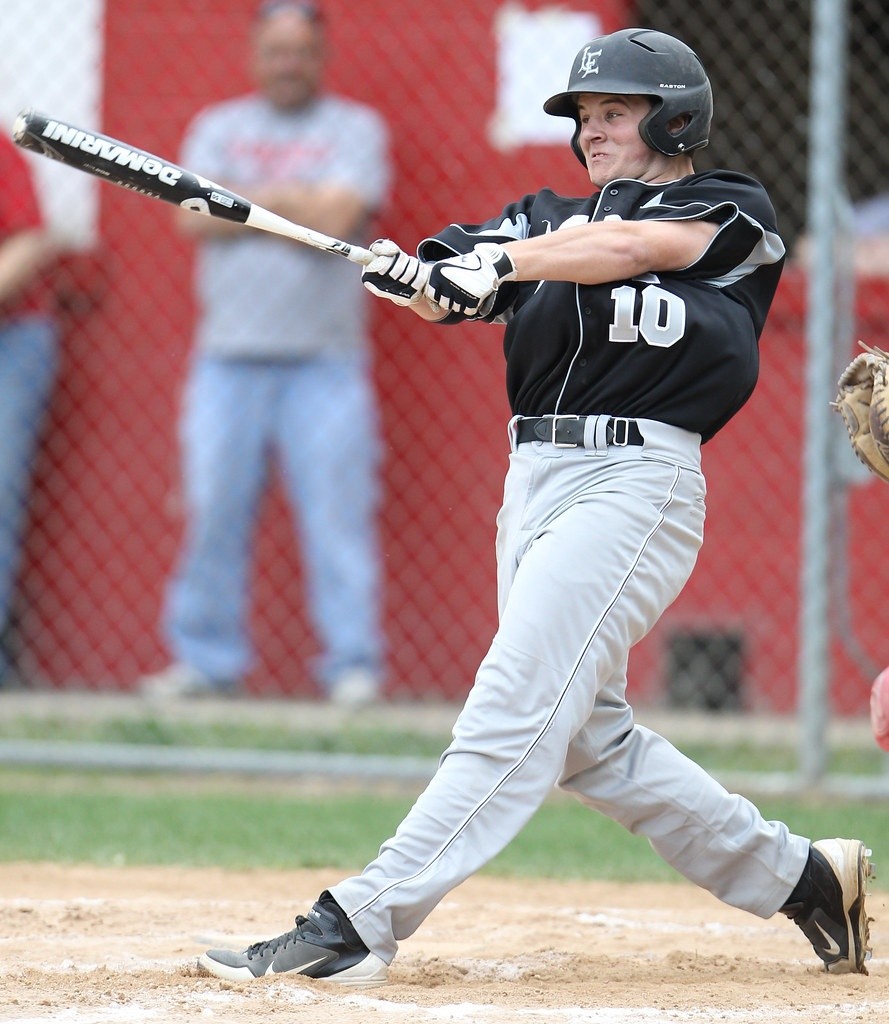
[517,417,644,446]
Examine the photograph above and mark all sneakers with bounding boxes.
[195,888,392,990]
[325,670,379,711]
[135,661,249,705]
[780,837,873,978]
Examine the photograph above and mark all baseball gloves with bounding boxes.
[825,339,889,483]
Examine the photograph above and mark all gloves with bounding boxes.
[362,239,425,307]
[426,241,515,319]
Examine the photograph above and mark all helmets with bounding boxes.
[544,26,712,167]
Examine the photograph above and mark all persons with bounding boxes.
[197,27,872,989]
[0,122,96,688]
[139,0,389,704]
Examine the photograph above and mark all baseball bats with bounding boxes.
[3,104,374,268]
